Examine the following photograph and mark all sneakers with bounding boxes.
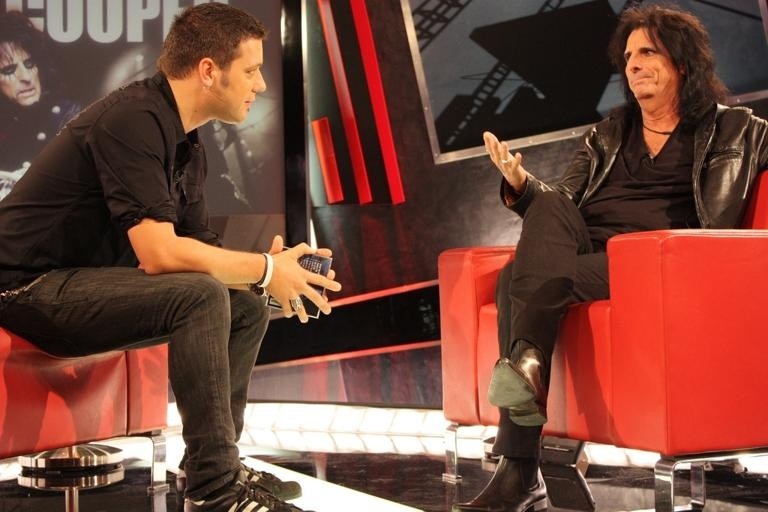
[176,464,302,503]
[184,471,315,512]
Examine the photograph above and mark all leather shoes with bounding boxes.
[488,338,549,427]
[451,454,548,511]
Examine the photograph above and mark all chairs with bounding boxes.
[0,319,172,466]
[435,167,768,512]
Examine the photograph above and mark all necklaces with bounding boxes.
[641,120,677,141]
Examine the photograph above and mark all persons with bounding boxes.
[454,4,768,511]
[1,10,88,178]
[1,2,345,512]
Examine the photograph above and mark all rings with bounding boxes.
[291,298,304,309]
[499,159,508,163]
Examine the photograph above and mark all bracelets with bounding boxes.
[256,252,274,288]
